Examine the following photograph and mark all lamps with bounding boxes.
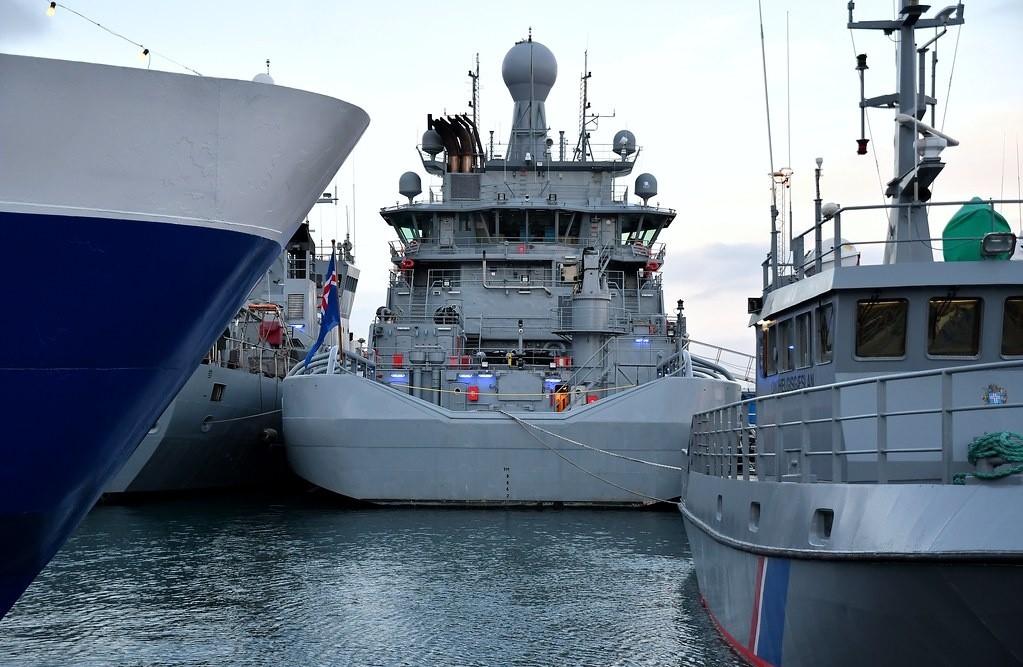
[137,48,150,63]
[46,0,56,18]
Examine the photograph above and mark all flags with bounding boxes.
[305,249,341,368]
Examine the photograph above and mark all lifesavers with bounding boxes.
[647,261,659,271]
[633,242,644,254]
[400,259,414,268]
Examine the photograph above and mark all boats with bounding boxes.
[85,51,363,500]
[0,49,371,623]
[275,31,744,507]
[679,0,1023,666]
